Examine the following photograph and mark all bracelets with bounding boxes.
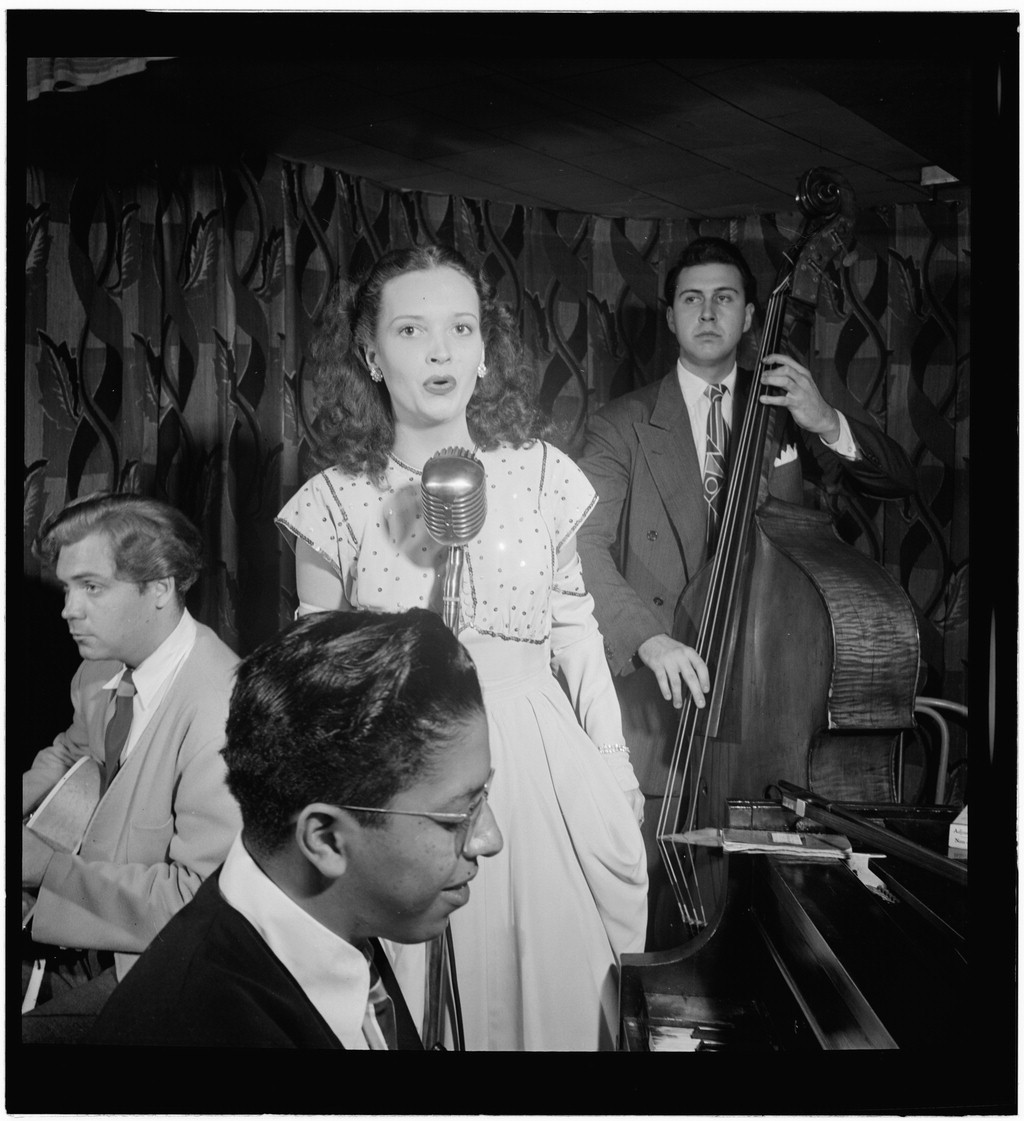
[597,743,632,755]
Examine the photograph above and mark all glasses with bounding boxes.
[283,770,498,860]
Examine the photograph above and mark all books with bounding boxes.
[722,827,853,859]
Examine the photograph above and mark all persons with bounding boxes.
[79,607,505,1051]
[23,491,243,1049]
[577,235,916,954]
[273,242,650,1051]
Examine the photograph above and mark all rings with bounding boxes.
[640,818,644,823]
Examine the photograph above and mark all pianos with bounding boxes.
[614,772,1016,1051]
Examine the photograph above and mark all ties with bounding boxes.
[704,385,732,558]
[371,978,398,1050]
[99,672,136,795]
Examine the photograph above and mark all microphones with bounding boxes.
[421,446,486,630]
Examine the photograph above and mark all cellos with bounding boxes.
[648,163,923,958]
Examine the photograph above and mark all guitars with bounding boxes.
[20,753,109,932]
